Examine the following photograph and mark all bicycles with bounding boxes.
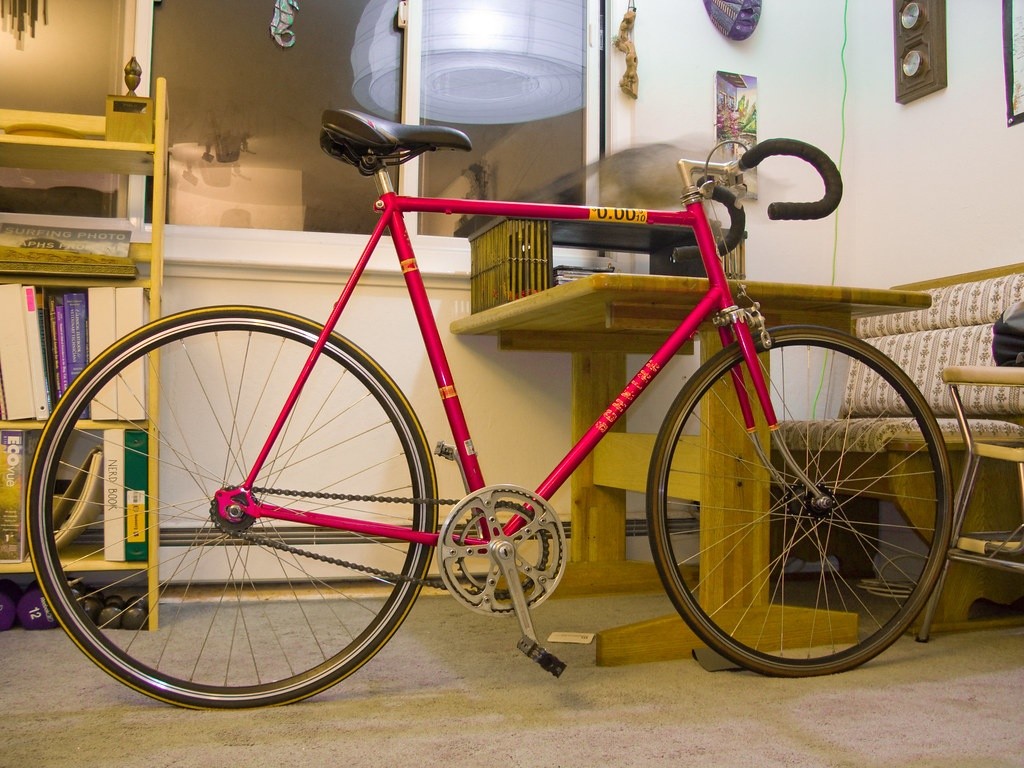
[22,105,956,714]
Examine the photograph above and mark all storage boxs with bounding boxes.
[453,213,748,317]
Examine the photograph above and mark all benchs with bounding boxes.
[771,261,1024,635]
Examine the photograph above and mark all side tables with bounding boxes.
[915,366,1024,643]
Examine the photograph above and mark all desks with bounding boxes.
[449,272,933,668]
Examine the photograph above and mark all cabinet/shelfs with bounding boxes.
[0,78,166,631]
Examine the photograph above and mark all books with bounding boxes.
[553,264,615,287]
[0,245,140,280]
[0,284,149,421]
[0,428,148,563]
